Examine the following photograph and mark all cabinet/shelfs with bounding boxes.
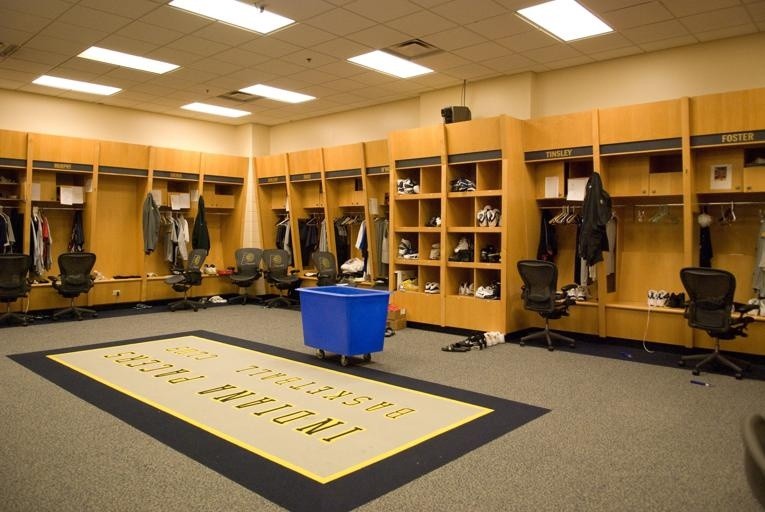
[269,177,364,210]
[203,183,235,209]
[607,157,684,198]
[692,149,764,194]
[153,180,192,208]
[535,159,593,200]
[388,113,531,342]
[33,171,87,205]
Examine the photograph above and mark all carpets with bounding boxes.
[5,329,554,512]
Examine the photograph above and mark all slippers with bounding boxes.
[384,326,395,338]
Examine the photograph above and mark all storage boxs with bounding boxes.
[294,285,390,365]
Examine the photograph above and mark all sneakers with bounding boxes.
[450,176,476,192]
[747,298,765,317]
[448,235,501,263]
[208,295,227,304]
[398,238,418,259]
[424,280,500,300]
[425,216,441,227]
[397,177,420,195]
[647,289,688,308]
[201,264,217,275]
[477,205,500,227]
[441,331,505,352]
[566,287,593,301]
[429,242,440,260]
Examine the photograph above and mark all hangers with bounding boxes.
[546,205,583,225]
[159,209,188,225]
[636,205,676,223]
[715,201,739,227]
[0,205,48,224]
[275,213,387,227]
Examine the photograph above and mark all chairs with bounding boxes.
[224,246,359,309]
[163,248,211,311]
[516,257,577,351]
[0,252,98,328]
[680,265,760,379]
